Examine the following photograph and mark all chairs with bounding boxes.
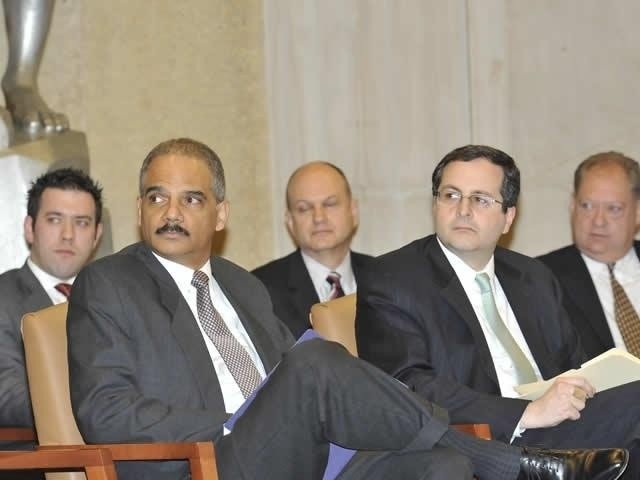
[20,300,219,480]
[310,292,490,440]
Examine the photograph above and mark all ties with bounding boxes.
[475,272,537,384]
[326,271,345,300]
[607,262,640,360]
[191,269,263,401]
[55,283,73,299]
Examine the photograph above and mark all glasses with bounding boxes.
[431,189,511,212]
[572,196,626,220]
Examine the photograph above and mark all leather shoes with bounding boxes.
[520,449,630,480]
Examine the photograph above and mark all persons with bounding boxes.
[0,167,103,428]
[249,160,378,342]
[529,153,640,358]
[65,137,629,479]
[354,145,640,480]
